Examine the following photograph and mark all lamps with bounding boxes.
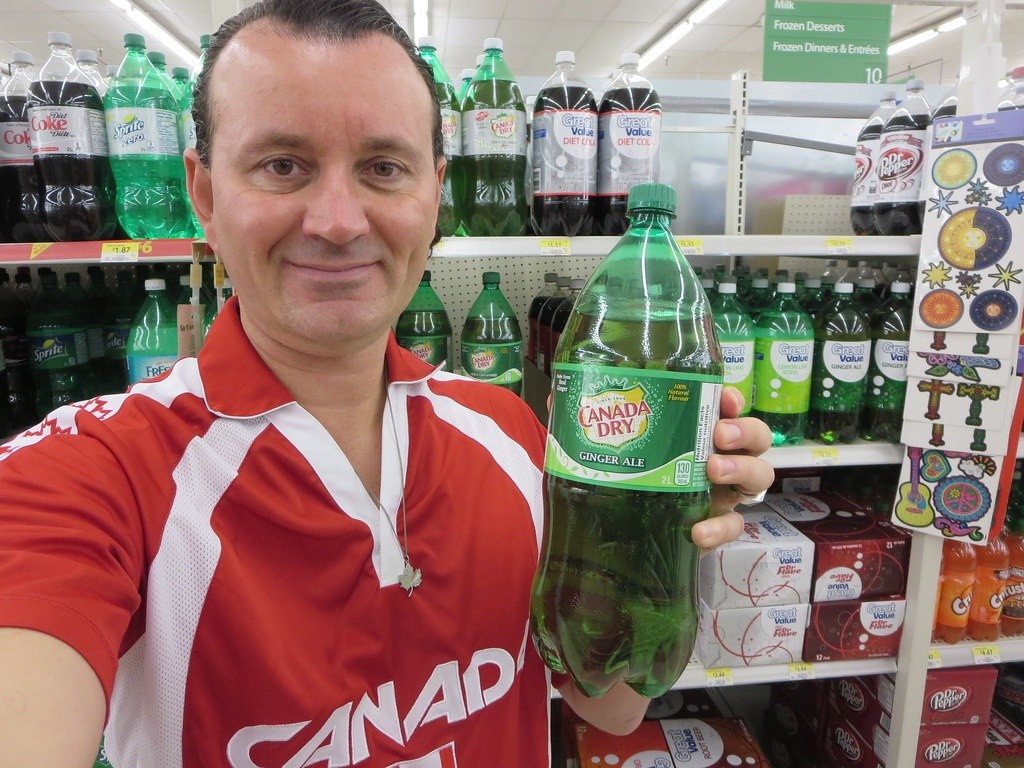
[633,0,727,71]
[412,0,429,53]
[109,0,200,69]
[887,10,968,57]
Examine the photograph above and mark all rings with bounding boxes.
[730,484,766,505]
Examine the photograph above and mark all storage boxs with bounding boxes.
[760,708,806,768]
[854,662,1002,719]
[822,699,886,768]
[766,694,825,768]
[645,688,737,720]
[569,718,676,768]
[661,716,772,768]
[765,490,912,602]
[829,677,990,768]
[700,497,812,610]
[692,599,807,667]
[770,679,827,729]
[803,597,909,666]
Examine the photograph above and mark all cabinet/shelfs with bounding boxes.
[0,75,1024,768]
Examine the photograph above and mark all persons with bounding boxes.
[0,0,775,768]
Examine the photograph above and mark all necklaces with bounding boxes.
[354,355,422,596]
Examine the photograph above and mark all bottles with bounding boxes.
[849,66,1024,235]
[0,31,662,242]
[527,183,723,701]
[0,259,915,448]
[930,459,1024,645]
